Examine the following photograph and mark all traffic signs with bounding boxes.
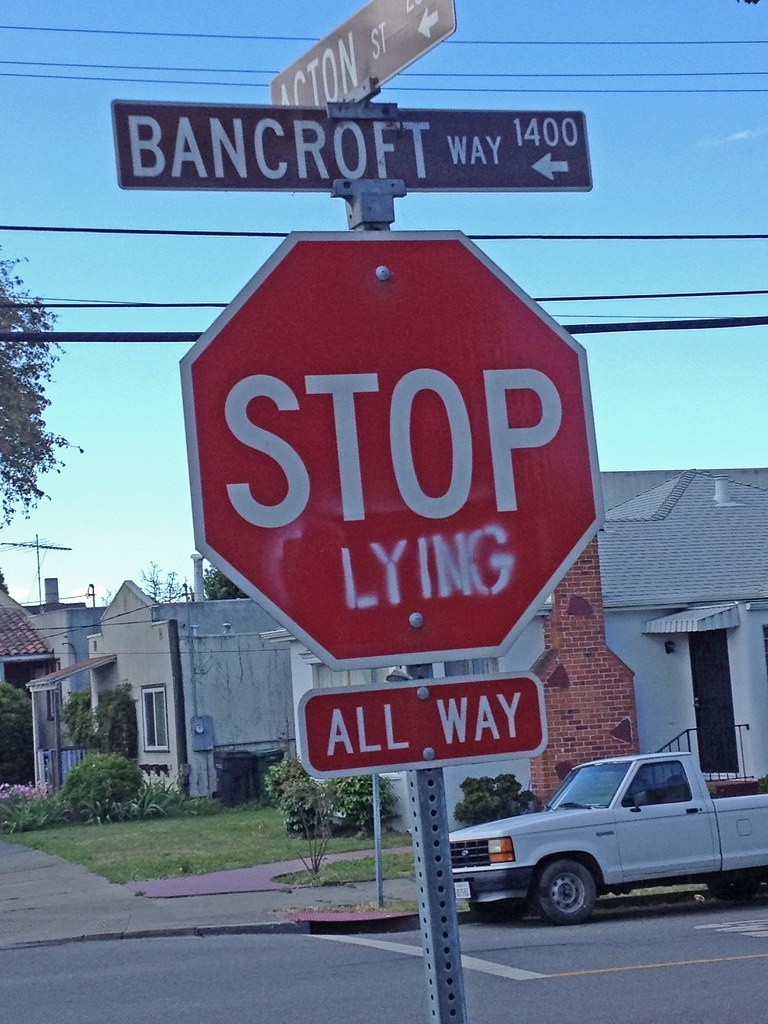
[111,99,593,192]
[270,1,457,105]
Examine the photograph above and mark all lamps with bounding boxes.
[664,641,675,655]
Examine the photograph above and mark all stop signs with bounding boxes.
[178,231,608,672]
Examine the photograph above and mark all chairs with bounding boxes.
[630,779,657,805]
[661,775,690,804]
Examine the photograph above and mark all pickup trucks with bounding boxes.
[449,752,768,927]
